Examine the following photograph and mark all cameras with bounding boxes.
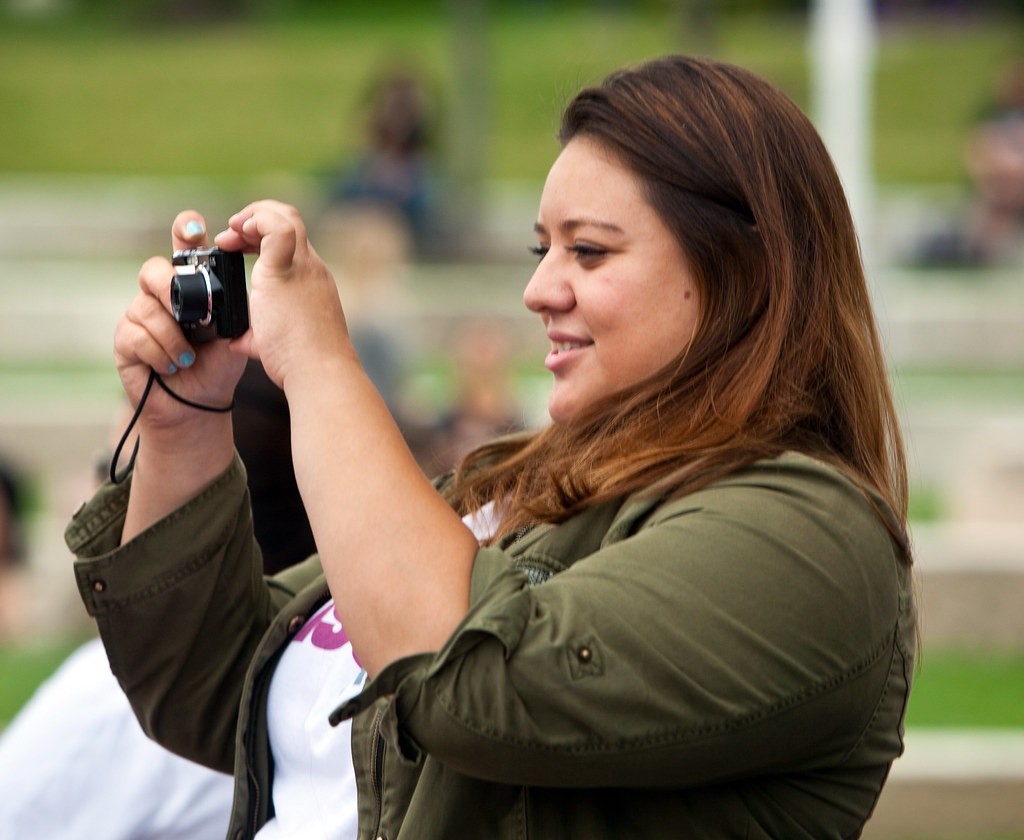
[170,246,249,341]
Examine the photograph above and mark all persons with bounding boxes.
[63,52,917,840]
[0,358,319,840]
